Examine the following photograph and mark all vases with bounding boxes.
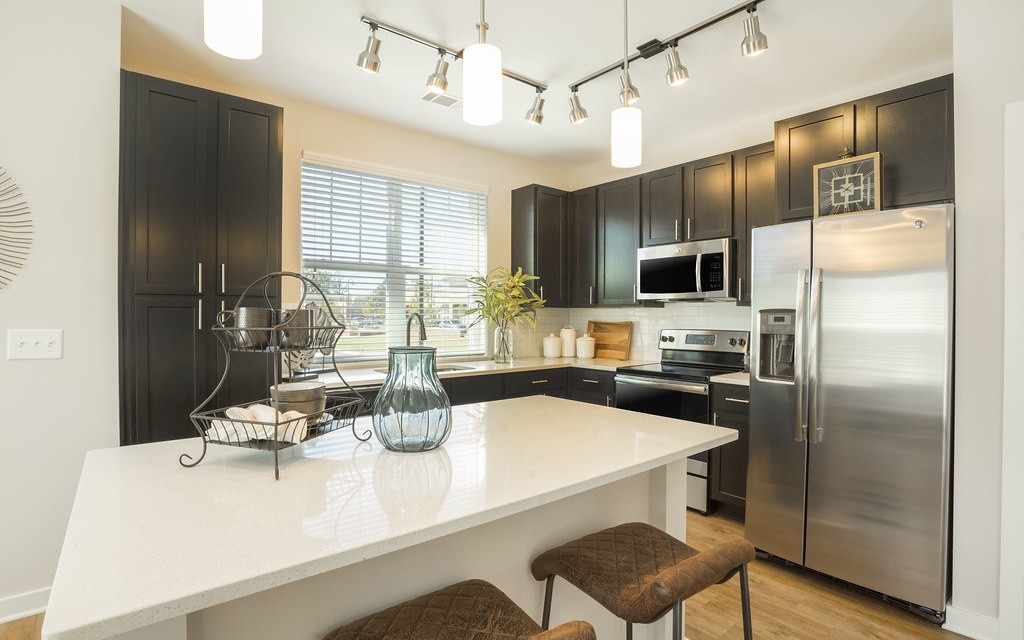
[494,327,513,364]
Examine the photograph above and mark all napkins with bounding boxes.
[206,404,307,446]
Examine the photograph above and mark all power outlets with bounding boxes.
[6,329,62,361]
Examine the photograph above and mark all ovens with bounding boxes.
[614,374,710,516]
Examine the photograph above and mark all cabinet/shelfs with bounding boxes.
[510,74,955,306]
[710,385,750,518]
[178,271,373,479]
[121,69,284,446]
[326,368,617,420]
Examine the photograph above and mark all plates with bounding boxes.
[307,413,333,430]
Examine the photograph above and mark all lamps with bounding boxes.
[568,85,589,123]
[664,38,689,86]
[611,0,642,168]
[618,61,642,105]
[426,48,450,95]
[461,0,503,127]
[524,85,546,125]
[203,0,262,60]
[741,1,768,58]
[356,22,382,72]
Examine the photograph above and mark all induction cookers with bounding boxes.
[616,329,750,384]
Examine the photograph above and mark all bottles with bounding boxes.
[372,346,452,452]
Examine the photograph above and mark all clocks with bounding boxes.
[813,152,883,220]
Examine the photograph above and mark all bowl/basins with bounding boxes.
[270,396,326,424]
[269,381,326,403]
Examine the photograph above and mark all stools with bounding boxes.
[320,579,596,640]
[531,522,755,640]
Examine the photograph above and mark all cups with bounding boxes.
[576,334,596,359]
[275,309,315,349]
[543,333,561,358]
[216,307,273,350]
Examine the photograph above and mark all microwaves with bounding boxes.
[637,238,737,300]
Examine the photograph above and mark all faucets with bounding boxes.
[406,312,427,347]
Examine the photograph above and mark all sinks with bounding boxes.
[374,365,476,375]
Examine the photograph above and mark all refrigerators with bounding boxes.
[744,203,954,624]
